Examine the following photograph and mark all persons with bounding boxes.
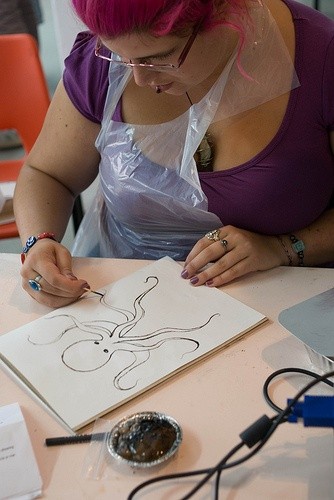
[13,0,334,309]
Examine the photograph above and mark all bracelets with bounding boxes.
[276,234,293,266]
[20,232,56,264]
[288,233,304,266]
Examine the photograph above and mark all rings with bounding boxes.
[28,275,42,290]
[220,239,228,252]
[206,229,220,242]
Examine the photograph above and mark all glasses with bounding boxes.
[94,14,209,71]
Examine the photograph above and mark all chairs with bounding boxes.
[0,32,51,239]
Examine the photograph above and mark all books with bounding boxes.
[0,255,267,436]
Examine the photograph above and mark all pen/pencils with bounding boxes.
[45,431,108,446]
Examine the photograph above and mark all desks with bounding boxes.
[0,252,334,500]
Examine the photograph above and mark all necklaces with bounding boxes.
[187,92,216,172]
